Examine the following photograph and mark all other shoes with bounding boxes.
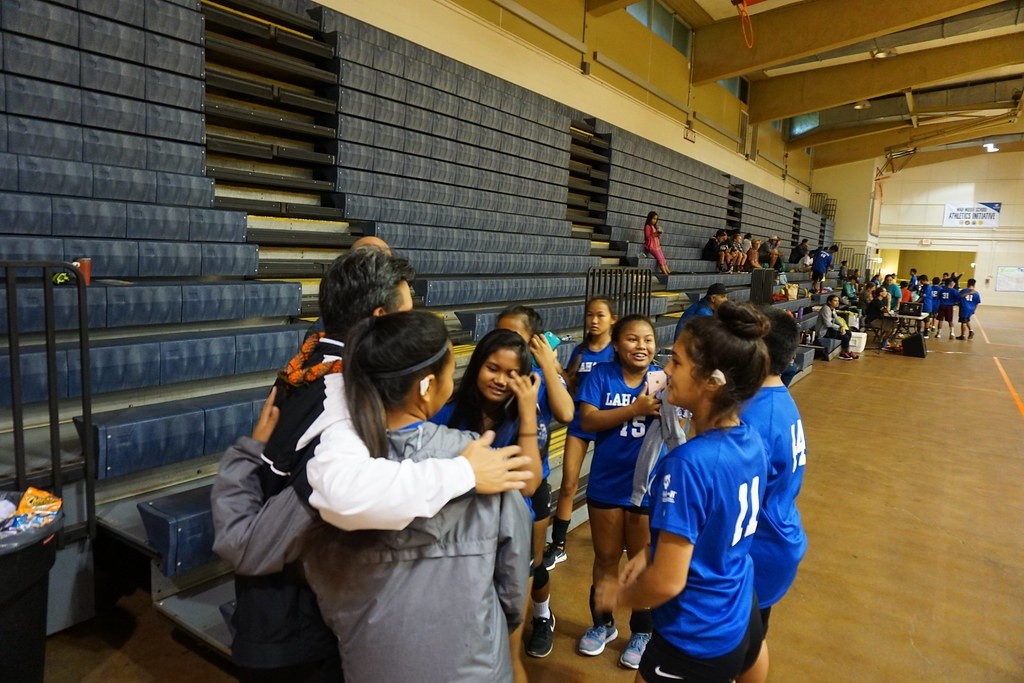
[820,290,830,294]
[955,333,967,341]
[923,331,930,339]
[935,332,942,338]
[968,331,975,341]
[949,332,955,339]
[879,346,890,350]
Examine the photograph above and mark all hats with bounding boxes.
[707,283,733,294]
[768,234,780,240]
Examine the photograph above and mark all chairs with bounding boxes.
[863,305,883,350]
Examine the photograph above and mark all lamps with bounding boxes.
[970,263,976,268]
[853,100,871,110]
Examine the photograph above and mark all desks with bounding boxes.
[884,310,929,341]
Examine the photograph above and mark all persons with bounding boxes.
[210,236,808,683]
[702,230,981,359]
[643,211,674,277]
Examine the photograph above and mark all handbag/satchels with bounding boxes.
[784,282,799,301]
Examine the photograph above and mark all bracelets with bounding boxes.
[518,433,537,437]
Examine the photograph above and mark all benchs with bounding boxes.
[0,154,861,668]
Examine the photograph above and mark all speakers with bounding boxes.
[901,332,927,358]
[750,267,775,307]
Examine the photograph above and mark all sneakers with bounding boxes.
[837,350,860,360]
[526,609,556,658]
[541,544,568,571]
[578,618,618,655]
[619,630,652,669]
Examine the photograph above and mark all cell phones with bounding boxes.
[528,331,563,368]
[646,370,668,397]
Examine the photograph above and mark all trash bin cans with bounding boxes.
[0,489,67,683]
